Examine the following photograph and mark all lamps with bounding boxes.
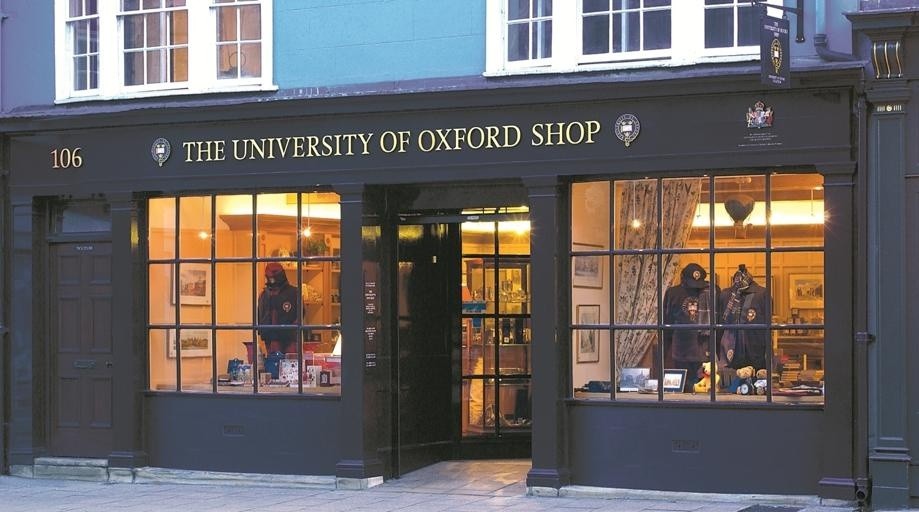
[723,178,755,240]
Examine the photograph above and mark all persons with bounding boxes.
[666,261,721,389]
[720,265,778,381]
[256,263,307,351]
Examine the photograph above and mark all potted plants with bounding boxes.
[301,237,328,267]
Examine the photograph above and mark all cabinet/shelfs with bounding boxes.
[259,228,340,353]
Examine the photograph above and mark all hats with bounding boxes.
[683,263,707,290]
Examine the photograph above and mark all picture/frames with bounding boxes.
[788,272,824,310]
[663,368,687,394]
[571,242,603,365]
[167,255,215,359]
